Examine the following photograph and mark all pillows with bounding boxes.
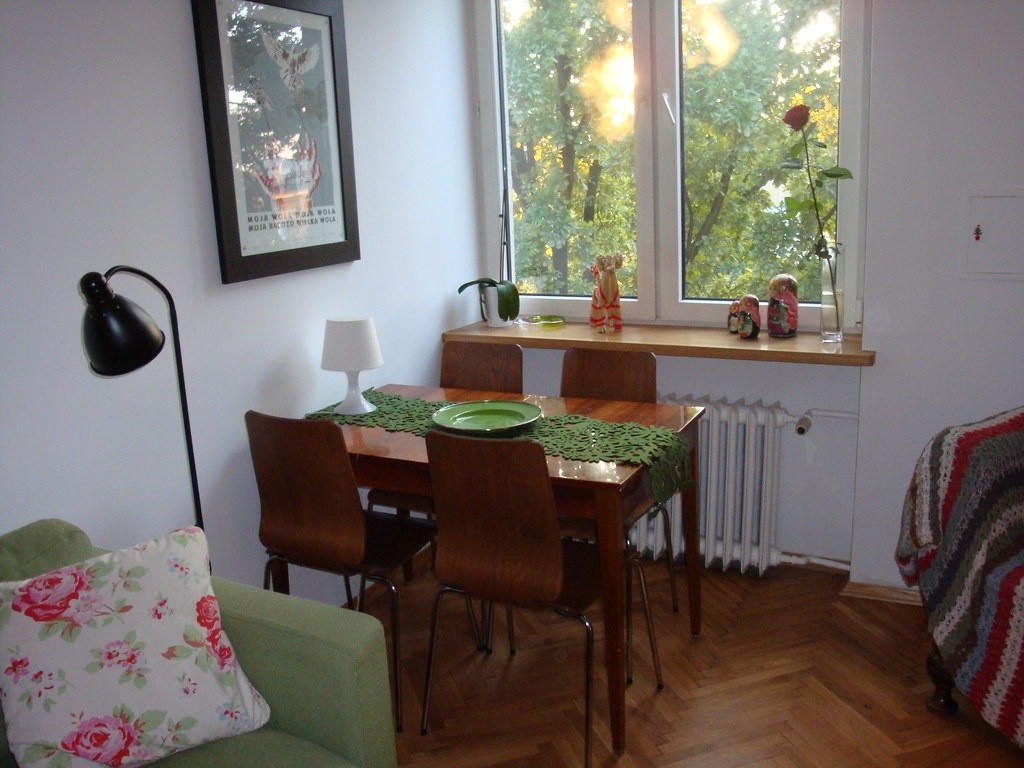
[0,524,272,768]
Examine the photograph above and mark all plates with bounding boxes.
[432,400,542,434]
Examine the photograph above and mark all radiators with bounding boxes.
[628,391,858,576]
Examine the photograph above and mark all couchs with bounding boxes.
[0,519,396,768]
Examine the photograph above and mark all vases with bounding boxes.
[818,246,846,344]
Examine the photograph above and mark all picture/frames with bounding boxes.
[192,0,361,283]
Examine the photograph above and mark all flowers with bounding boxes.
[591,254,623,284]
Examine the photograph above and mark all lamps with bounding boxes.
[780,104,854,332]
[79,265,212,576]
[320,316,384,415]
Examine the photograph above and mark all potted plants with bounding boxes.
[457,278,520,328]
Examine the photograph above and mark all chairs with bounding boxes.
[244,410,480,734]
[504,349,679,686]
[420,428,664,768]
[355,341,524,649]
[894,403,1024,751]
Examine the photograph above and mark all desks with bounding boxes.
[443,318,874,367]
[300,386,706,757]
